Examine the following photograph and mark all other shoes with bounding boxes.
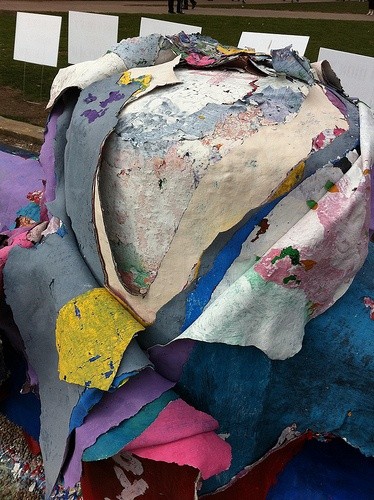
[192,1,197,9]
[182,5,189,10]
[167,9,186,15]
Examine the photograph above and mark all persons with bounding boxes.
[167,0,185,14]
[182,0,197,9]
[365,0,374,16]
